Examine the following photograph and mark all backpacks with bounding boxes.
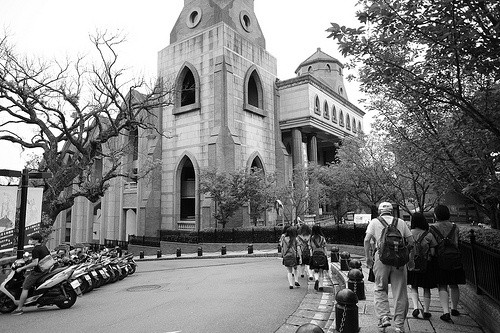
[376,216,410,269]
[430,224,464,281]
[298,236,310,264]
[310,236,327,269]
[282,236,296,267]
[407,229,430,271]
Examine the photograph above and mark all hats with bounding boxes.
[378,201,393,214]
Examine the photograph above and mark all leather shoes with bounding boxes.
[451,309,460,316]
[423,312,431,319]
[440,313,450,321]
[289,273,319,290]
[412,309,419,317]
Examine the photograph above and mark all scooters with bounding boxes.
[0,242,137,317]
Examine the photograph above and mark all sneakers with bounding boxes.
[395,326,405,333]
[10,309,24,315]
[377,316,391,328]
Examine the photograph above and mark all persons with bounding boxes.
[429,204,461,321]
[403,212,439,320]
[281,225,301,289]
[279,226,295,277]
[363,201,415,333]
[296,224,314,279]
[309,224,329,293]
[11,232,51,315]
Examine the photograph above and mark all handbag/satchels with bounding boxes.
[37,245,55,272]
